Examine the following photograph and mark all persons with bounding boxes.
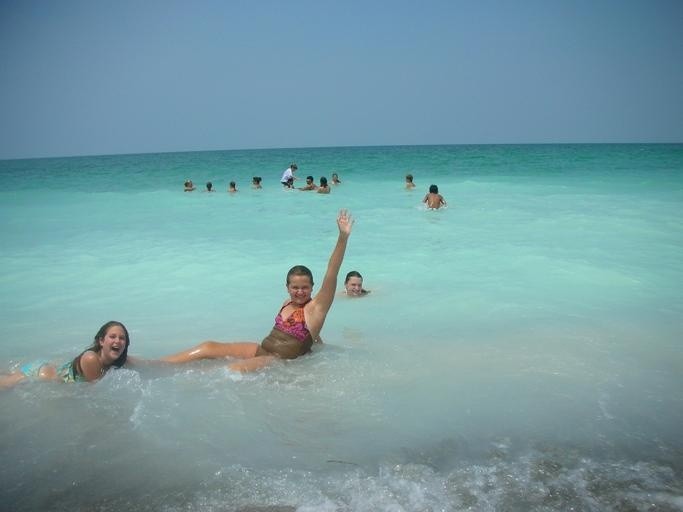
[204,182,217,192]
[317,177,330,193]
[280,163,298,189]
[404,174,416,188]
[183,178,197,191]
[251,176,263,189]
[423,185,446,210]
[301,175,318,192]
[125,208,356,375]
[331,173,342,184]
[227,181,238,192]
[0,319,129,394]
[337,271,370,298]
